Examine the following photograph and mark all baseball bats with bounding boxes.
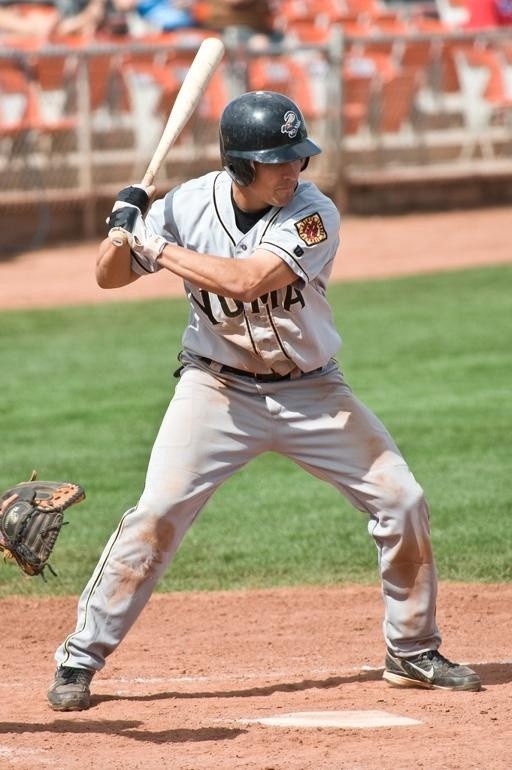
[110,37,224,246]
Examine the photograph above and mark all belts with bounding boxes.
[197,353,325,385]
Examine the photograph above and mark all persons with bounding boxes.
[0,0,286,80]
[47,88,481,711]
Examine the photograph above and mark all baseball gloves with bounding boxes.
[0,480,85,575]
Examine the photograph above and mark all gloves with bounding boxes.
[103,182,173,265]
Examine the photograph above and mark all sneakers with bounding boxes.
[379,644,484,695]
[43,664,94,713]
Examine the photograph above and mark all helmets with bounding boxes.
[216,88,324,189]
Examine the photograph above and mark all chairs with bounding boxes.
[1,1,512,193]
